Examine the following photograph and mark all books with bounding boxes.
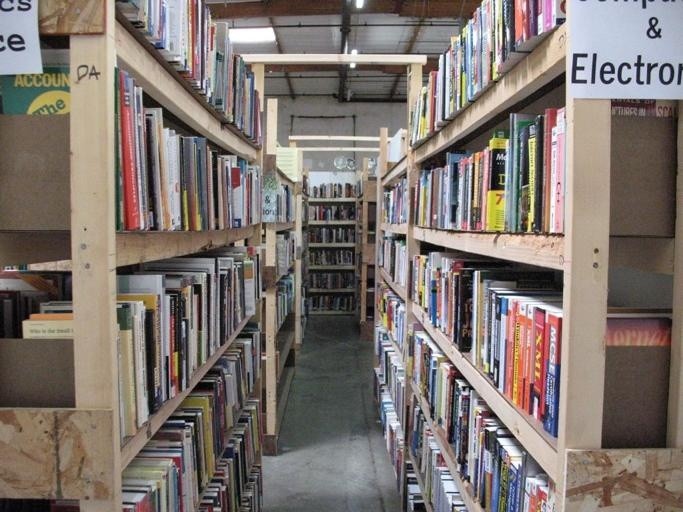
[369,1,683,512]
[0,0,300,511]
[301,167,370,324]
